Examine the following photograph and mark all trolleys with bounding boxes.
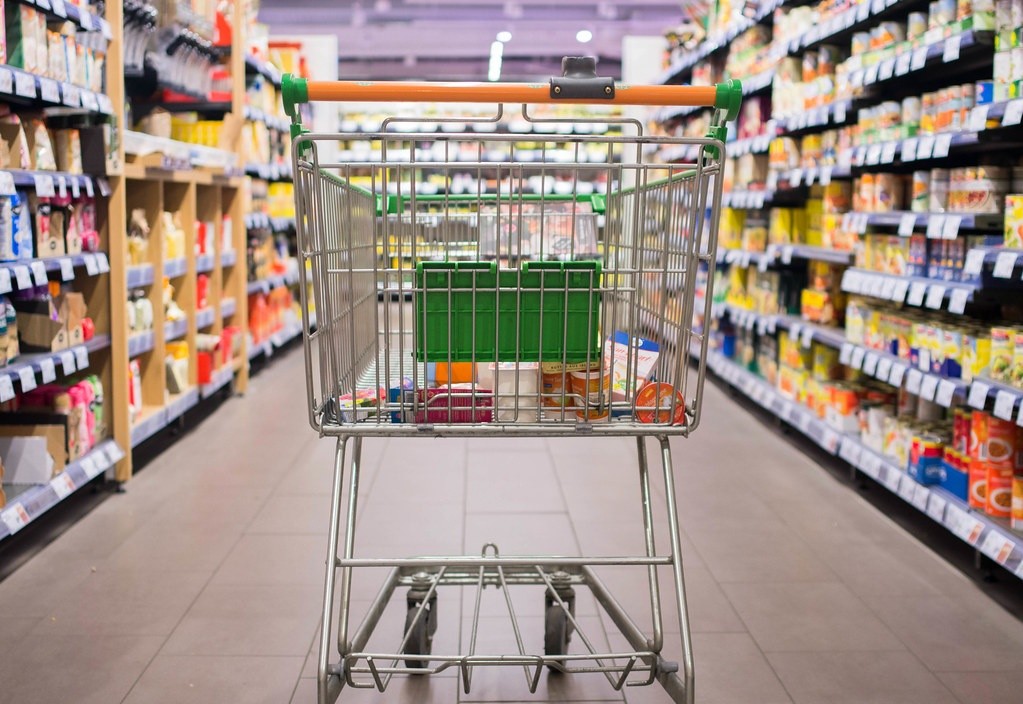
[278,52,745,704]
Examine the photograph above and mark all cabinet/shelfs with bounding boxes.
[0,1,1022,585]
